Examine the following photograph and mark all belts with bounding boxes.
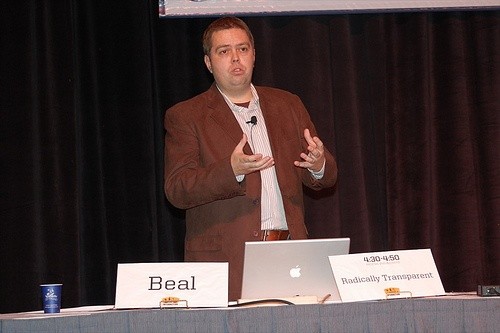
[262,229,291,241]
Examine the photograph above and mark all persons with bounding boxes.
[164,15,338,300]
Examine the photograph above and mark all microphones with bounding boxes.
[246,115,259,153]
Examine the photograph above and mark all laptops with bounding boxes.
[228,237,351,305]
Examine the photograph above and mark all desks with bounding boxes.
[1,291,500,333]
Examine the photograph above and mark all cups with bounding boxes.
[39,284,64,313]
